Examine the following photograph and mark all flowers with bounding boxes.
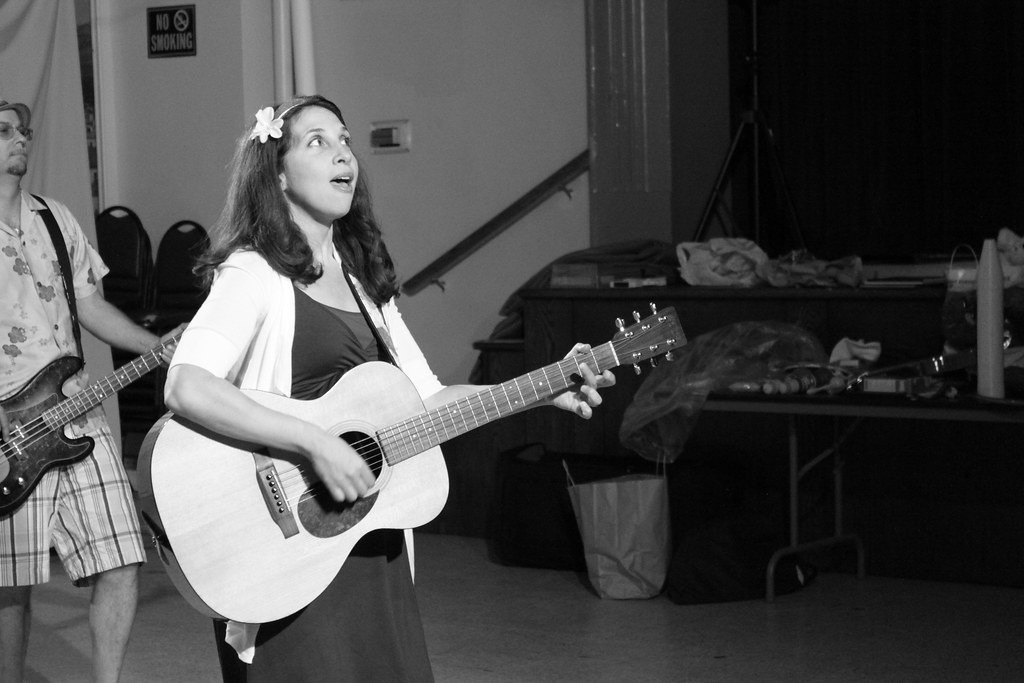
[248,106,283,143]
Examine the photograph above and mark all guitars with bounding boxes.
[136,303,687,624]
[0,325,186,516]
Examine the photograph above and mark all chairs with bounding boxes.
[93,206,214,444]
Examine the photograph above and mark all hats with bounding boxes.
[0,99,31,130]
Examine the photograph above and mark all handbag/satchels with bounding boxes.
[486,442,569,569]
[677,237,768,287]
[562,447,669,599]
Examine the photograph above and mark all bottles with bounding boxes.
[764,366,834,395]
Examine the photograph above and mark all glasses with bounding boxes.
[0,125,33,141]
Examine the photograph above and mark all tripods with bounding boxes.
[695,0,804,253]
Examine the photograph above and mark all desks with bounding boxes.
[649,390,1024,604]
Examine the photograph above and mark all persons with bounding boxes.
[0,99,191,683]
[164,96,616,682]
